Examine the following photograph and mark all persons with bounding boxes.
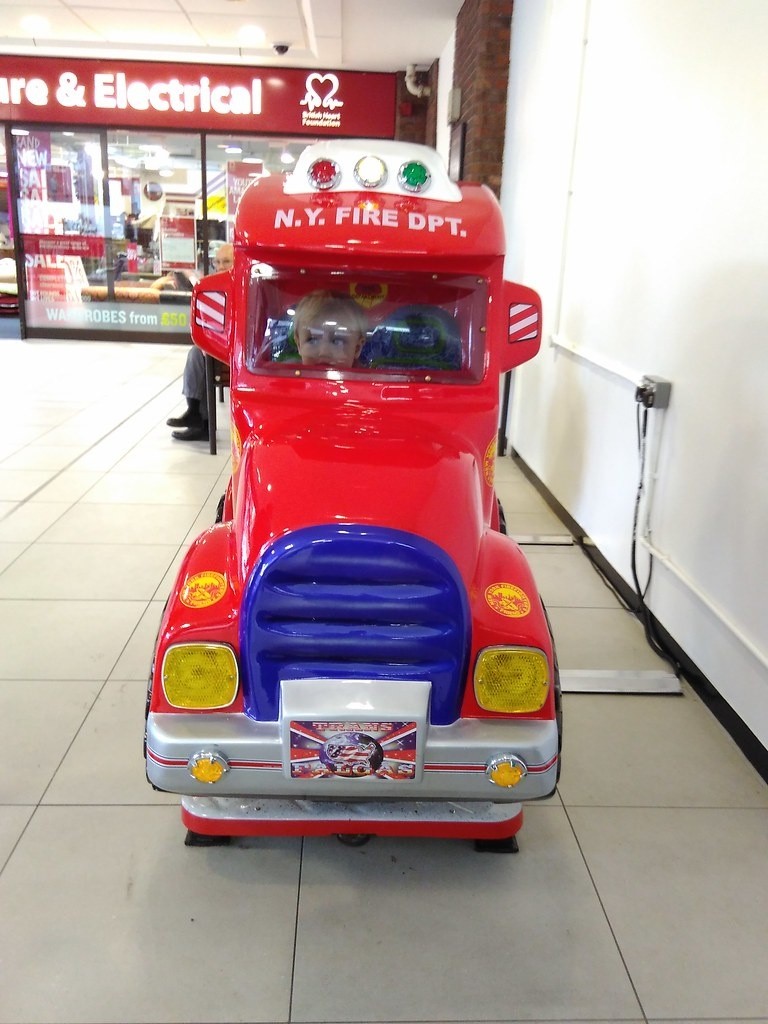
[165,242,235,440]
[284,290,370,374]
[123,214,139,272]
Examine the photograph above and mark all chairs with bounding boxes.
[357,303,464,370]
[268,302,304,367]
[203,351,230,455]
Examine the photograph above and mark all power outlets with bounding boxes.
[643,375,671,409]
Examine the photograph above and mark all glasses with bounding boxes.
[210,260,231,267]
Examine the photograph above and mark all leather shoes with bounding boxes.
[166,408,201,427]
[171,426,208,440]
